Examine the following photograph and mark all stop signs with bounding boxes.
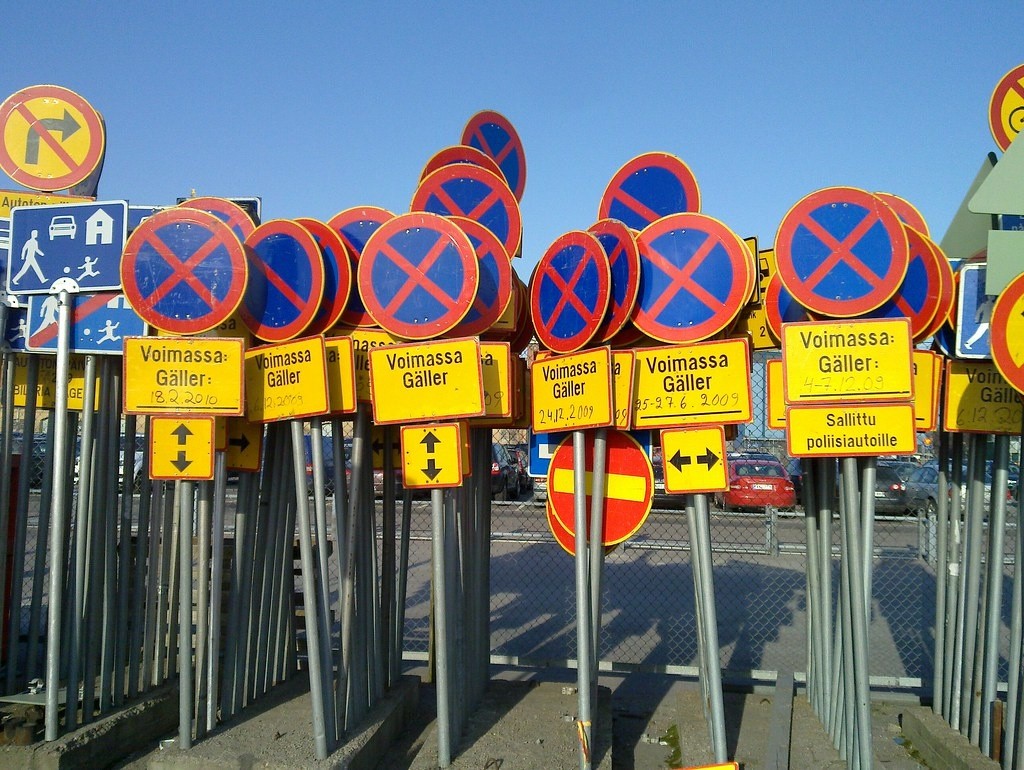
[548,428,655,558]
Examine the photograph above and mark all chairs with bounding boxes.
[738,466,749,474]
[767,468,779,475]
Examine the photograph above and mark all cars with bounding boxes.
[789,459,1018,522]
[0,431,147,495]
[260,433,412,501]
[650,446,687,510]
[716,451,797,513]
[489,441,548,501]
